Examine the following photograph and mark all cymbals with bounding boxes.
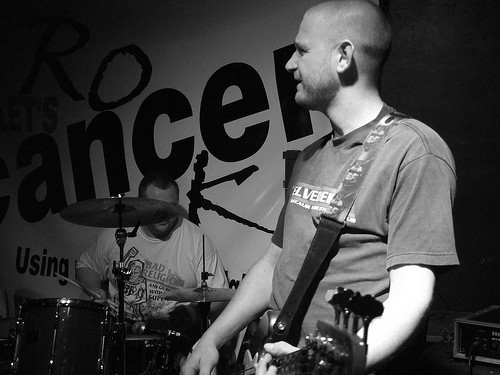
[159,287,236,301]
[61,198,180,228]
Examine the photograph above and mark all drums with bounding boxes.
[110,336,172,375]
[14,299,106,375]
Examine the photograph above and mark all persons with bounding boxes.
[76,172,229,318]
[180,0,462,375]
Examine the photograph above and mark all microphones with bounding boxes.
[132,322,181,338]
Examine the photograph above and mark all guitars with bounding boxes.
[219,321,384,375]
